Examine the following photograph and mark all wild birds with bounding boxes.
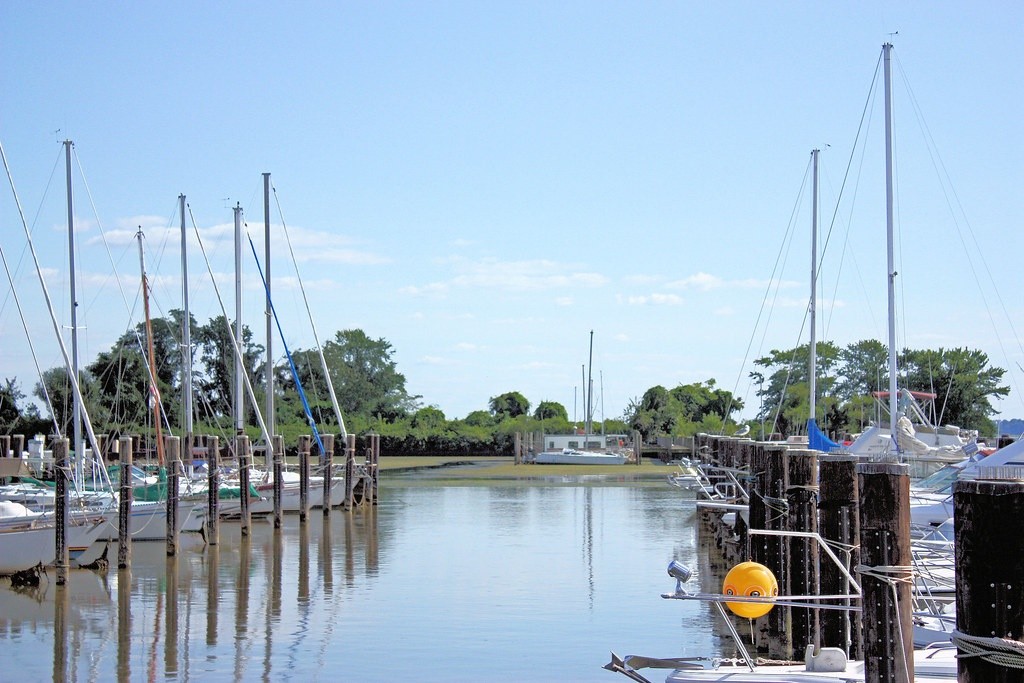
[732,424,751,438]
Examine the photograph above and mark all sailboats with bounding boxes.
[0,135,368,576]
[535,329,629,467]
[757,41,1023,542]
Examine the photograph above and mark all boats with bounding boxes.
[600,532,959,682]
